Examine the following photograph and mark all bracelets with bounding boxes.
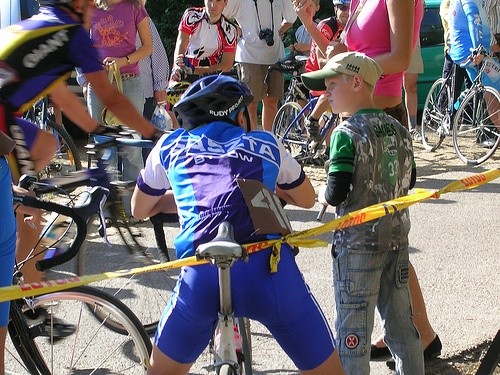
[125,55,130,66]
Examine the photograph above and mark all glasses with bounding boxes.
[338,5,349,11]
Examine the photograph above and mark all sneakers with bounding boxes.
[14,299,77,339]
[410,125,428,142]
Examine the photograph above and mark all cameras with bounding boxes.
[259,28,274,46]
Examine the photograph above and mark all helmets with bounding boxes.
[333,0,351,6]
[167,82,193,106]
[174,74,255,130]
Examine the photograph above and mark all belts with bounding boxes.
[111,73,137,81]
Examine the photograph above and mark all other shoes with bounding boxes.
[370,341,391,360]
[304,113,322,142]
[386,334,442,368]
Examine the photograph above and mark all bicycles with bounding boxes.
[7,55,252,375]
[421,44,500,165]
[157,197,286,375]
[265,60,345,168]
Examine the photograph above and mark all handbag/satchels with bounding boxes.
[102,60,130,127]
[150,100,174,138]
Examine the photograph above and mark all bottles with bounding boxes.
[319,110,331,127]
[454,88,469,109]
[232,326,243,355]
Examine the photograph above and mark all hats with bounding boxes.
[300,51,384,91]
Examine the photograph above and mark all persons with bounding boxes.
[294,1,442,372]
[78,0,500,221]
[300,52,424,375]
[131,75,347,375]
[0,0,175,375]
